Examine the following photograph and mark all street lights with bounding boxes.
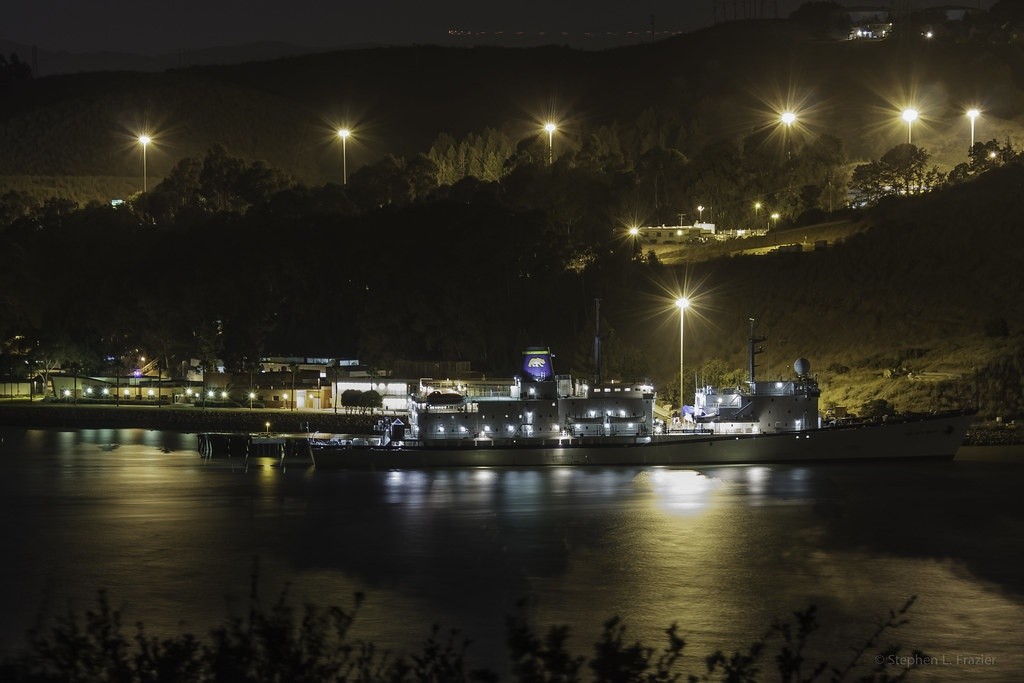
[138,135,151,192]
[779,109,797,161]
[966,108,980,161]
[697,206,705,223]
[544,122,558,164]
[755,203,761,222]
[900,106,919,145]
[337,129,350,186]
[674,295,693,421]
[771,213,779,228]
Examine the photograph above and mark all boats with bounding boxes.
[303,407,981,465]
